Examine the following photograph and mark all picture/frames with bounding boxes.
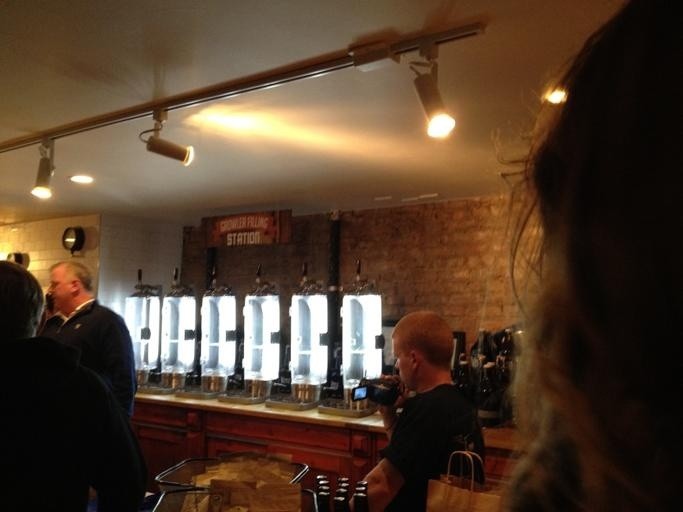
[383,320,400,374]
[450,331,464,381]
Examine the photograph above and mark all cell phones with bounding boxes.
[44,291,54,308]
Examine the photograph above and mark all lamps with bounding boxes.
[0,21,484,199]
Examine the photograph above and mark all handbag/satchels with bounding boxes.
[427,450,506,512]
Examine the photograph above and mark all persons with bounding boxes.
[349,308,484,511]
[494,1,683,511]
[38,259,136,422]
[0,259,149,512]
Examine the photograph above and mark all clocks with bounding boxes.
[62,226,85,254]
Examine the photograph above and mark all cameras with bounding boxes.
[350,376,399,407]
